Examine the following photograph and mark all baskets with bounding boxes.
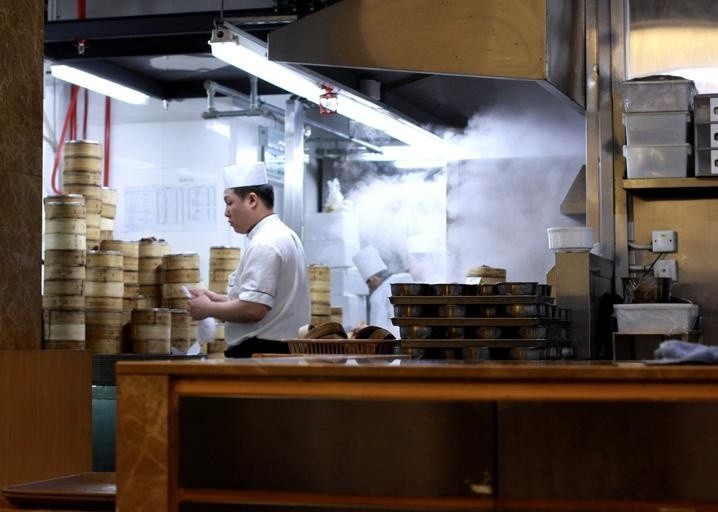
[287,338,401,355]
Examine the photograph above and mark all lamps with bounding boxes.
[207,0,446,151]
[50,64,150,106]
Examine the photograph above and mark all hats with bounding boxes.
[351,243,388,283]
[221,161,268,189]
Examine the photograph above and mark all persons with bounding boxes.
[351,245,414,340]
[186,161,312,358]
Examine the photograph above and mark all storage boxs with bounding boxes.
[623,144,691,179]
[621,110,687,144]
[624,80,691,111]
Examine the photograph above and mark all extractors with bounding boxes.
[267,1,587,148]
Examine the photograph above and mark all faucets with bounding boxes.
[625,281,636,304]
